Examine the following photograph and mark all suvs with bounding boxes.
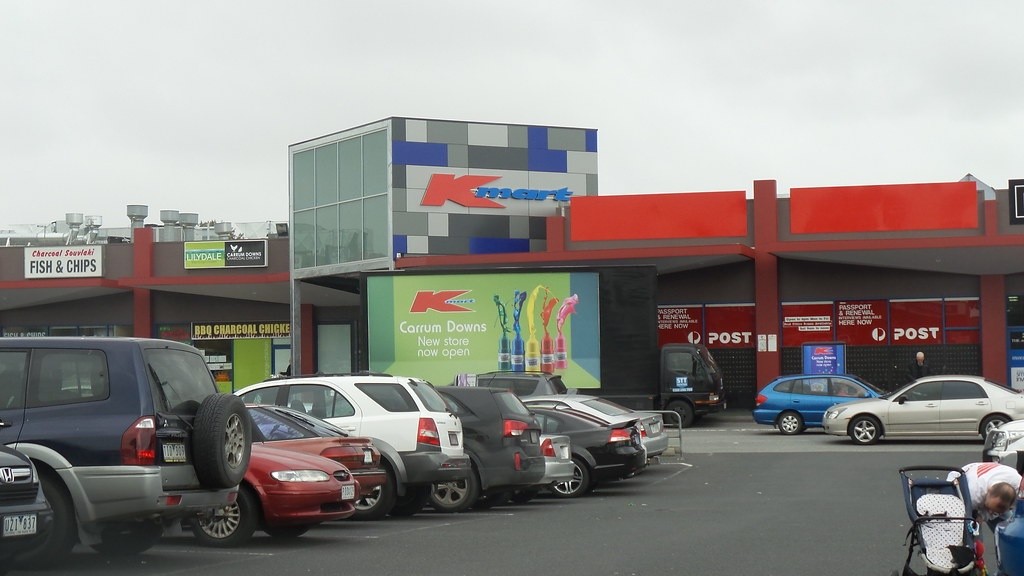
[234,372,473,521]
[0,336,253,556]
[447,371,567,397]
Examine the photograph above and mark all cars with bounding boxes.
[0,444,53,563]
[526,406,646,497]
[243,401,385,501]
[984,419,1024,477]
[188,449,359,549]
[822,375,1024,446]
[520,395,671,458]
[994,478,1024,576]
[752,372,885,435]
[514,434,573,502]
[427,384,546,514]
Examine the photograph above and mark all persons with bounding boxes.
[949,461,1024,541]
[279,358,291,377]
[905,352,928,384]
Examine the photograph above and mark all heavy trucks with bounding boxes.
[657,342,726,428]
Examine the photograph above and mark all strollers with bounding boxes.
[898,464,985,576]
